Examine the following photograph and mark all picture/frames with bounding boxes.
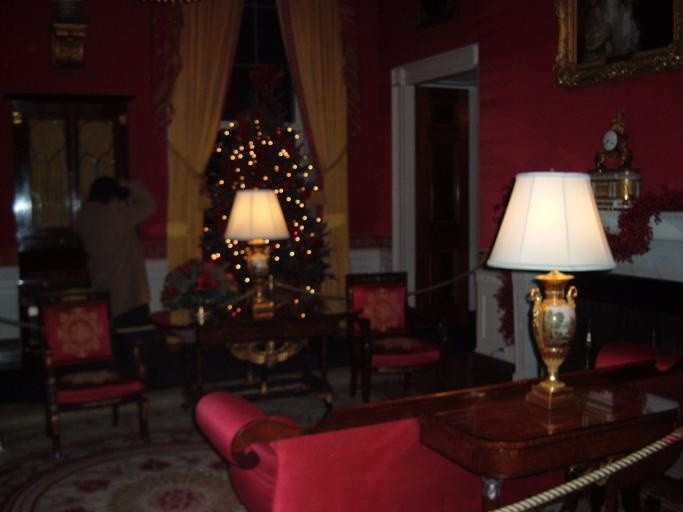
[550,1,682,93]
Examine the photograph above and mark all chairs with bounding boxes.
[342,271,446,407]
[34,288,157,463]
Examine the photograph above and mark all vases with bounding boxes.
[193,307,218,328]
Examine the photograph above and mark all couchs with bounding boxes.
[191,357,676,512]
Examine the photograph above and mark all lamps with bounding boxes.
[223,187,292,324]
[479,170,619,412]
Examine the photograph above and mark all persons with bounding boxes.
[75,174,171,392]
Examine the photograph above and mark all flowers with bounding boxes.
[159,258,232,309]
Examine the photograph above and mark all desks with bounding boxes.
[150,294,365,434]
[414,368,682,512]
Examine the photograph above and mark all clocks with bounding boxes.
[587,109,640,211]
